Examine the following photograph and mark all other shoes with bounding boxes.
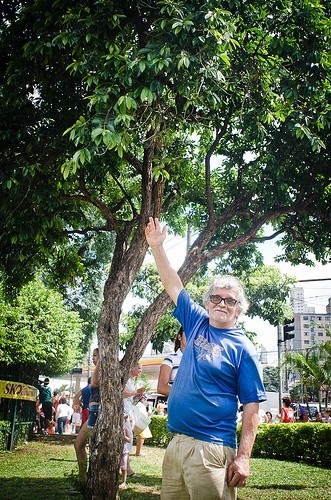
[127,468,135,476]
[119,483,128,489]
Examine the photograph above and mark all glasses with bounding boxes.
[209,295,240,307]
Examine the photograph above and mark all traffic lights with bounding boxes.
[284,317,295,342]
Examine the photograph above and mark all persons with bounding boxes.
[145,217,267,500]
[75,377,91,429]
[118,412,131,490]
[74,348,101,488]
[145,399,168,418]
[134,395,153,456]
[236,397,331,424]
[122,362,141,478]
[157,326,186,398]
[33,378,83,435]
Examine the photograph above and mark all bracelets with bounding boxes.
[79,404,82,406]
